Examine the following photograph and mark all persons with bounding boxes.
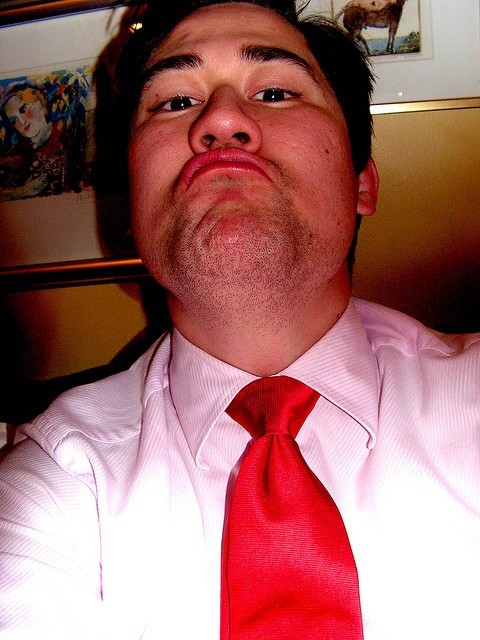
[0,81,67,158]
[0,1,480,640]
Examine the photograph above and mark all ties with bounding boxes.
[220,377,364,635]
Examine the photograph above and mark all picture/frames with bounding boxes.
[0,0,154,290]
[295,0,480,117]
